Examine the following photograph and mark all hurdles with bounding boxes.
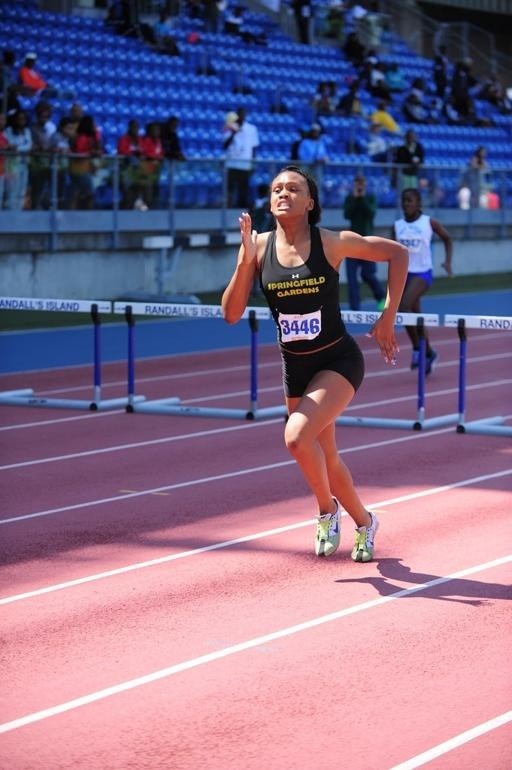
[113,300,290,421]
[0,297,147,414]
[331,310,460,432]
[444,313,512,437]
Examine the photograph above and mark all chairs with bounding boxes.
[0,0,512,209]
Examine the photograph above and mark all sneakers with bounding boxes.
[315,497,342,556]
[412,346,436,373]
[351,512,379,562]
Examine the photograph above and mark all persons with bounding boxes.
[341,175,387,310]
[390,188,452,375]
[219,165,410,564]
